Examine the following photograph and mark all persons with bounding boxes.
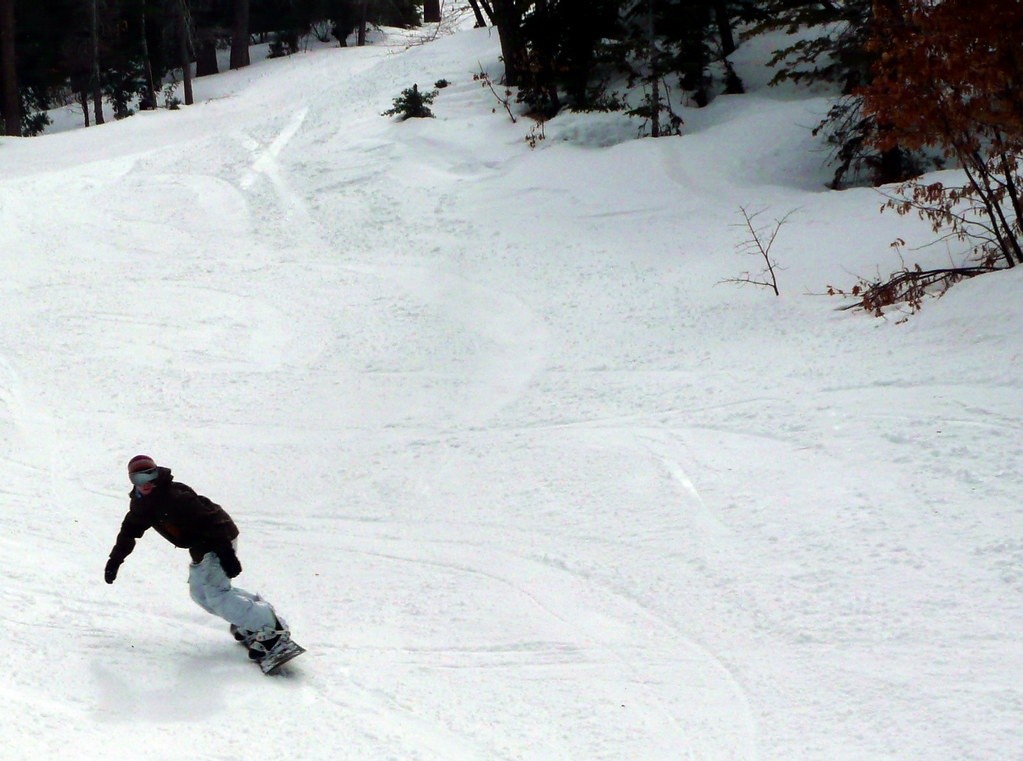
[105,455,289,664]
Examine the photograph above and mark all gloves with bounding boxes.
[217,546,242,579]
[104,554,125,584]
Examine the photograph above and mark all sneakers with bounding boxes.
[234,612,286,660]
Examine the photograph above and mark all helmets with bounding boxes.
[128,455,159,486]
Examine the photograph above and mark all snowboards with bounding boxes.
[229,612,306,676]
